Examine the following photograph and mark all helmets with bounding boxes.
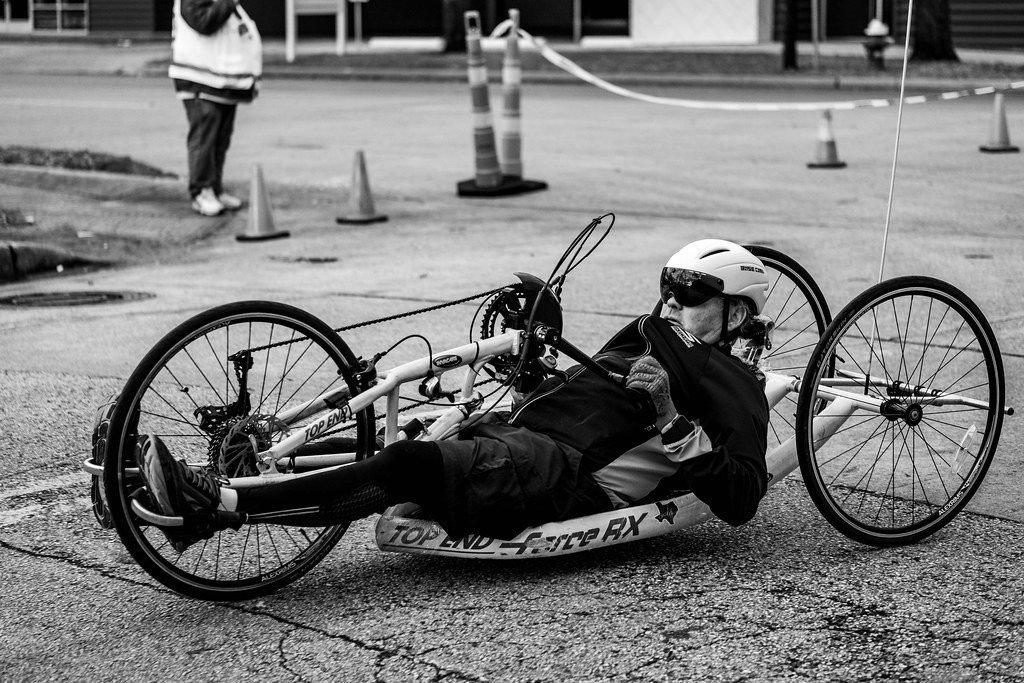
[659,238,770,316]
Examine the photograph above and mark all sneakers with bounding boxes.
[216,191,243,210]
[91,392,158,530]
[190,186,227,217]
[133,433,230,552]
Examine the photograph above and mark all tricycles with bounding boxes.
[90,213,1015,603]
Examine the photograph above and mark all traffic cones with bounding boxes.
[805,109,846,169]
[336,150,392,228]
[237,164,293,242]
[977,92,1020,155]
[455,9,549,199]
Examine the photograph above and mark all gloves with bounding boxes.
[626,356,678,431]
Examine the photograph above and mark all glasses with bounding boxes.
[660,267,739,308]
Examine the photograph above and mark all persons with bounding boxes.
[168,0,263,216]
[91,239,771,550]
